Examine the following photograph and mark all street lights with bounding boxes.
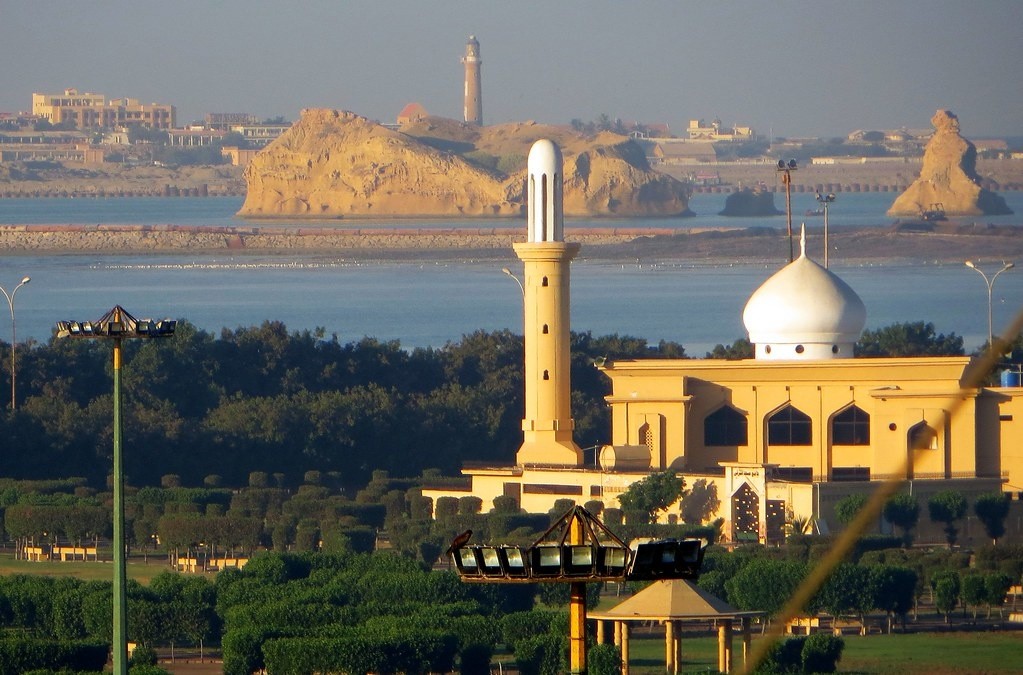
[776,158,799,263]
[0,277,32,411]
[54,304,179,675]
[449,504,633,674]
[965,261,1014,387]
[814,189,836,270]
